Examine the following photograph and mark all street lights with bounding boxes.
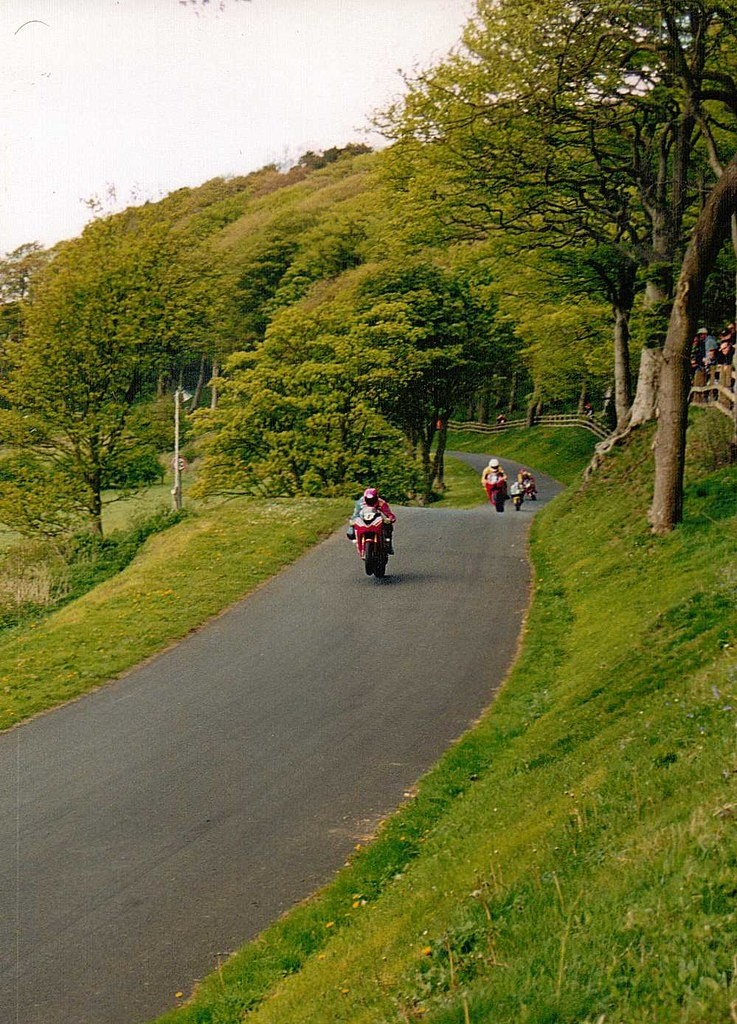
[172,388,194,509]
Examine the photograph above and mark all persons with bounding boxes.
[497,411,508,430]
[583,404,594,429]
[482,458,509,500]
[349,488,395,557]
[512,468,536,504]
[691,322,737,413]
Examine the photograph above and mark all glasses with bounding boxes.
[491,466,498,469]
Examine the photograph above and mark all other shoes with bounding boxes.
[388,549,394,555]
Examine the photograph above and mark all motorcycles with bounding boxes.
[510,479,539,511]
[349,505,393,579]
[484,472,507,512]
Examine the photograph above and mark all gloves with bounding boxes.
[384,519,391,525]
[350,521,354,527]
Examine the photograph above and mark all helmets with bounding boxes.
[364,489,377,500]
[489,459,499,467]
